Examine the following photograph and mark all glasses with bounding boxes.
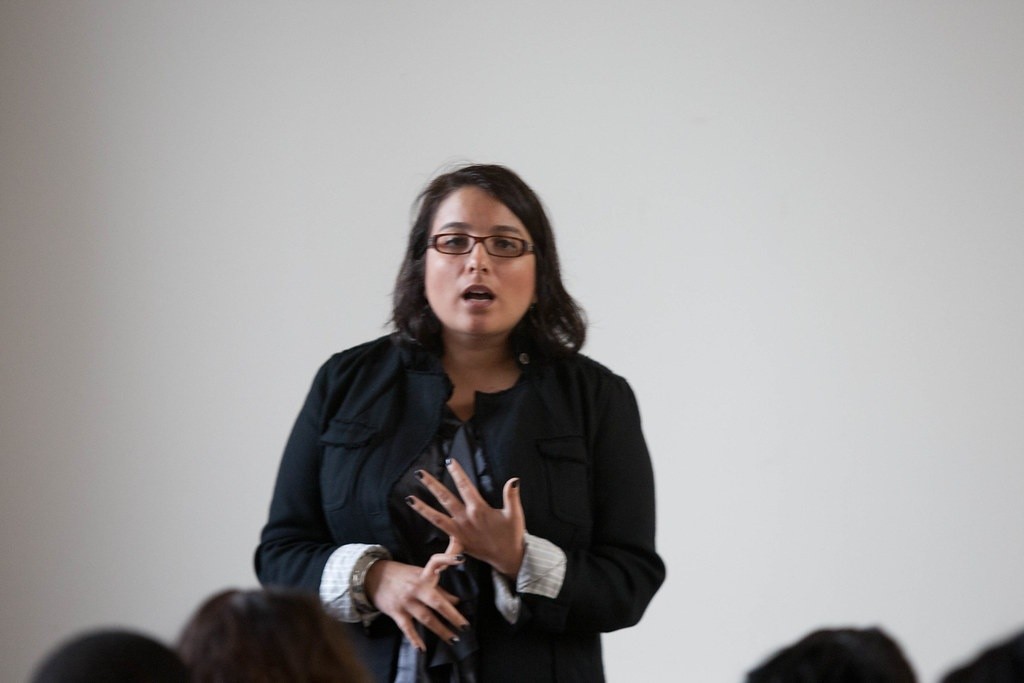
[424,232,536,258]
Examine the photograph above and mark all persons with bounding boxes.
[31,630,189,683]
[177,589,372,683]
[252,165,667,683]
